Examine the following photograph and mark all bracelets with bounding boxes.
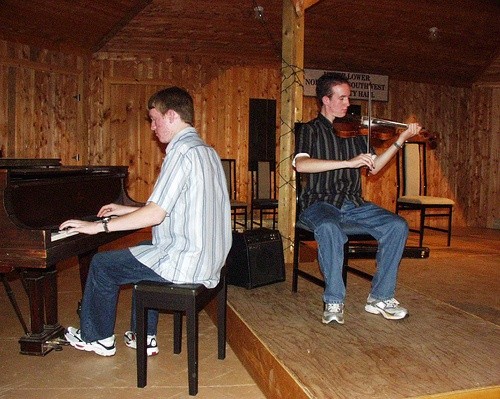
[394,142,402,150]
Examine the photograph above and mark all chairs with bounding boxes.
[291,122,379,311]
[395,141,456,248]
[221,159,248,231]
[251,158,278,229]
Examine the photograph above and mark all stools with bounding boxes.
[135,261,228,396]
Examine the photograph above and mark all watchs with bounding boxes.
[102,218,111,233]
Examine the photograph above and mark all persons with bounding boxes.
[289,72,422,324]
[59,87,233,356]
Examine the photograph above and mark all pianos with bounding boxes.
[0,158,147,357]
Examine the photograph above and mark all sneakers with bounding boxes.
[365,295,409,320]
[322,301,345,325]
[63,326,117,356]
[124,330,159,357]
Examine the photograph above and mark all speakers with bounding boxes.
[247,98,277,161]
[226,227,286,288]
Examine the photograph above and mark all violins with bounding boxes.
[333,112,440,151]
